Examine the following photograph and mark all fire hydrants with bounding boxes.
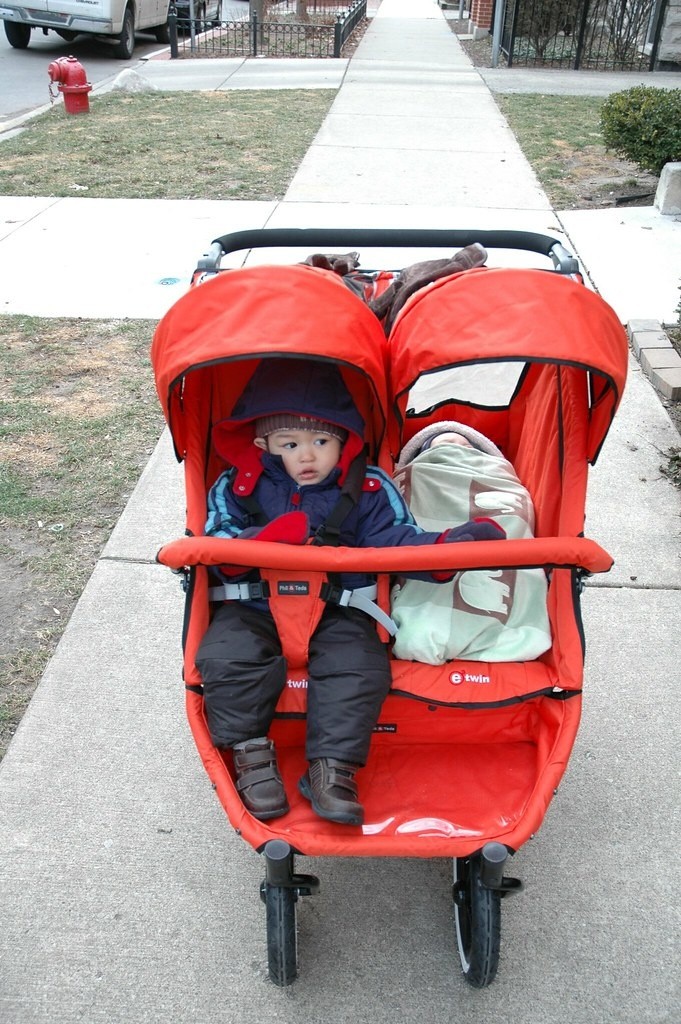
[47,54,93,115]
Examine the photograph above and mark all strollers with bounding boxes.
[150,223,629,991]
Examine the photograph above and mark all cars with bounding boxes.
[173,0,225,36]
[0,0,180,60]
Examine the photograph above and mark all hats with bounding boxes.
[420,430,480,454]
[254,414,349,443]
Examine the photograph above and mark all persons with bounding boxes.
[420,431,476,453]
[192,357,507,824]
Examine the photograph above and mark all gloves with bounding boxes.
[219,512,310,577]
[432,518,506,582]
[298,251,360,277]
[368,241,488,339]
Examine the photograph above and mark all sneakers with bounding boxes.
[232,737,289,818]
[298,756,364,825]
